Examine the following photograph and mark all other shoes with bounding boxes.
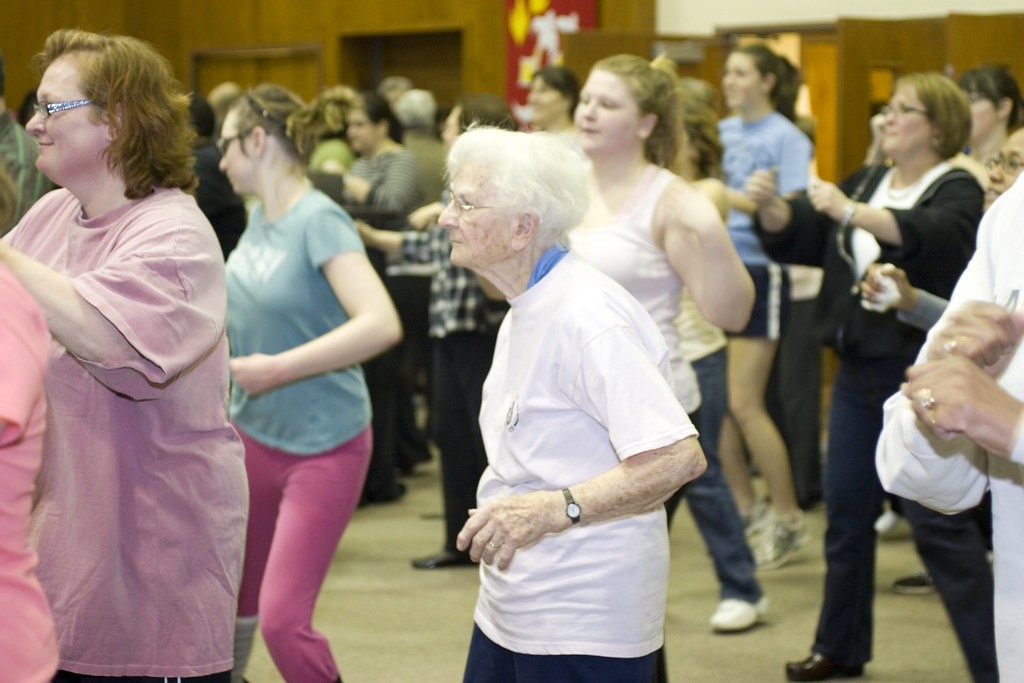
[874,510,901,535]
[412,548,477,568]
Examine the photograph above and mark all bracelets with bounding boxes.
[841,201,856,226]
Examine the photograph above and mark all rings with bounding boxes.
[920,395,935,409]
[489,541,495,548]
[944,341,956,352]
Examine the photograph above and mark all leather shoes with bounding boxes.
[785,651,863,681]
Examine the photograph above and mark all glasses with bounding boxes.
[986,154,1023,174]
[878,104,931,118]
[966,90,995,103]
[447,188,502,218]
[32,98,97,120]
[214,129,270,155]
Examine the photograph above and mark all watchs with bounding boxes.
[563,488,581,524]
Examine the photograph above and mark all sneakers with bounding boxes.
[753,508,810,571]
[710,594,767,632]
[894,565,939,594]
[737,497,773,537]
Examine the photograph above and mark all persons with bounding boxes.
[0,27,1024,683]
[437,125,709,683]
